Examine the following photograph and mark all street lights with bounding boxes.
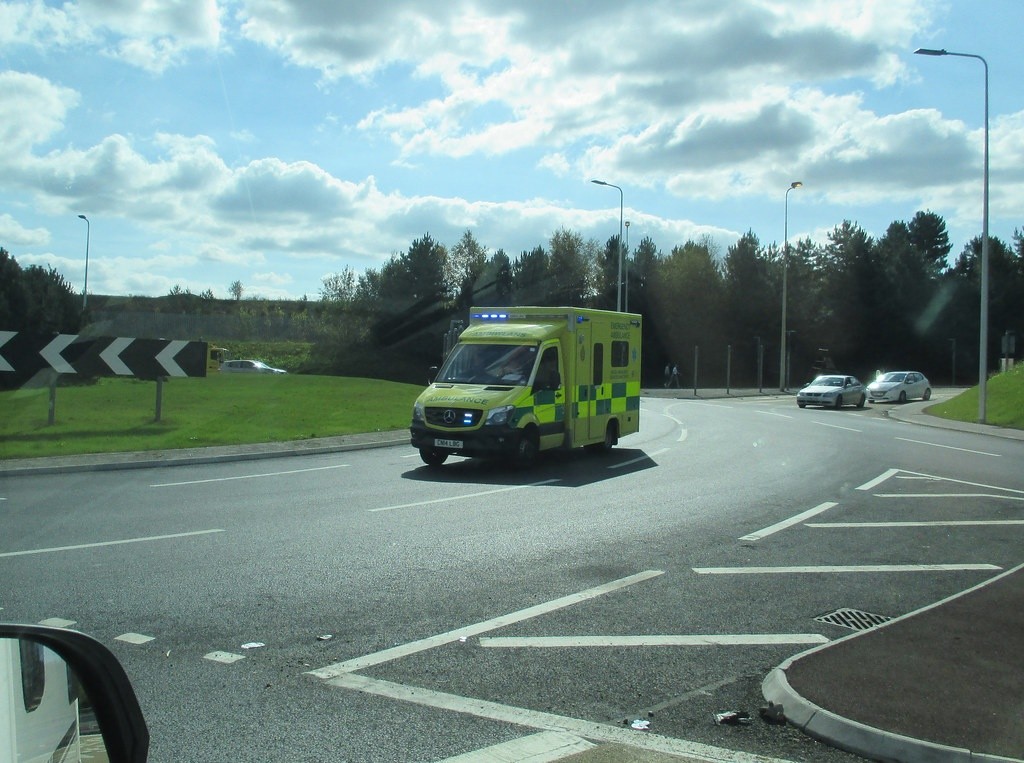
[914,47,990,423]
[785,329,796,392]
[77,214,90,311]
[947,337,956,385]
[625,221,631,314]
[590,178,623,314]
[779,181,803,393]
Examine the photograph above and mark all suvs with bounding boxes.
[223,359,289,374]
[209,346,231,373]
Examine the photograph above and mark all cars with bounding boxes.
[865,372,932,404]
[796,375,867,411]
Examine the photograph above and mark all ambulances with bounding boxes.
[409,306,642,474]
